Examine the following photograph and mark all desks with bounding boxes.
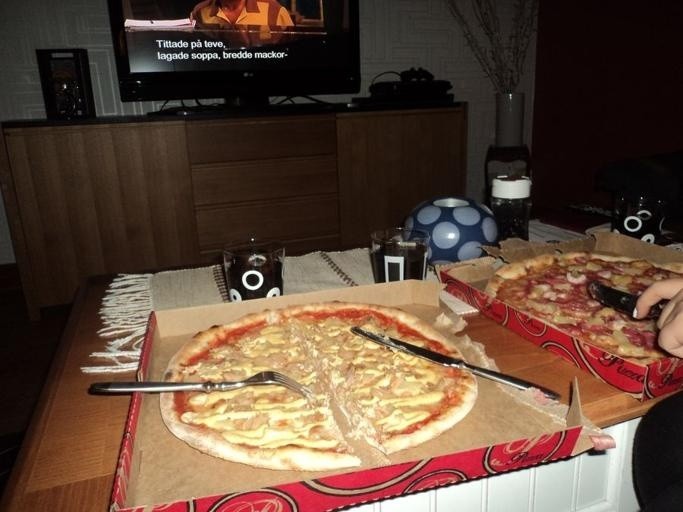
[2,205,683,511]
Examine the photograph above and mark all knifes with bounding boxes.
[589,283,662,319]
[351,324,560,401]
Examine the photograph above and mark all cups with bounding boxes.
[222,238,286,301]
[614,193,667,246]
[490,175,532,240]
[368,225,431,282]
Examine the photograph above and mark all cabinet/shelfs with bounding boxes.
[0,101,469,322]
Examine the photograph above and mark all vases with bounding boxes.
[484,92,532,211]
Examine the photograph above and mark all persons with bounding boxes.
[633,278,683,512]
[190,0,294,26]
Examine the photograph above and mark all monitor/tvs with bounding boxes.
[108,0,361,118]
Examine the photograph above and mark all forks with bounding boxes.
[89,371,313,400]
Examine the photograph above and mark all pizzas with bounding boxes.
[482,250,683,367]
[159,298,480,474]
